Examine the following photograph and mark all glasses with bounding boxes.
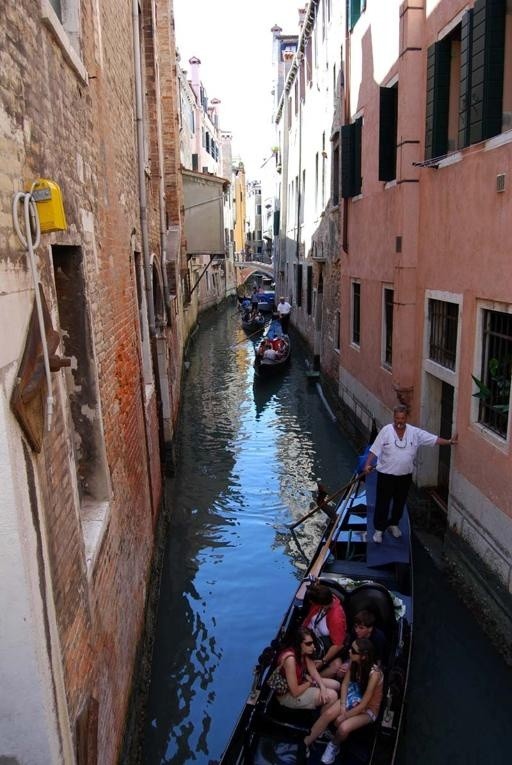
[350,644,359,655]
[303,640,314,646]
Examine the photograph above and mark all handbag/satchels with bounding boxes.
[267,669,289,697]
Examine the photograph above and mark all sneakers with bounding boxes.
[387,525,403,538]
[301,729,340,764]
[372,529,383,543]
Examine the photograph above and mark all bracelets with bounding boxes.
[449,439,451,445]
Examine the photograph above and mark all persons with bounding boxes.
[274,585,384,765]
[363,406,460,544]
[242,285,292,360]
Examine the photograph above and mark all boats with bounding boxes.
[239,274,291,366]
[217,417,415,765]
